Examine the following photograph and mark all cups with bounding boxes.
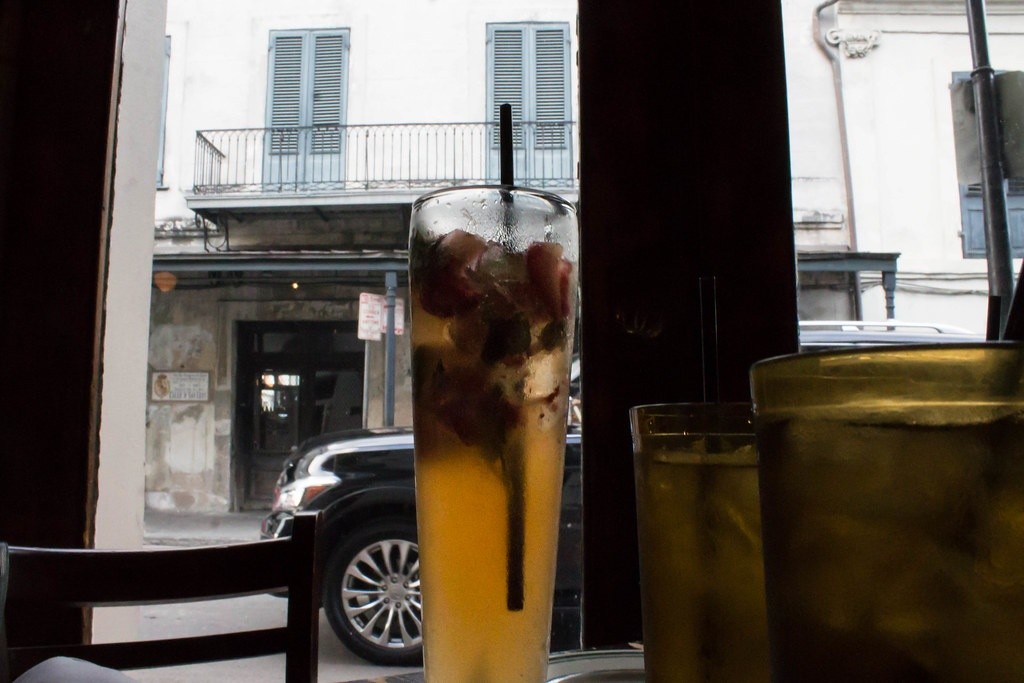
[748,339,1023,682]
[405,186,578,683]
[629,399,771,682]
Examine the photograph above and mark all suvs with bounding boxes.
[257,319,984,670]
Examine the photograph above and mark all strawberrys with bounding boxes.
[411,230,575,445]
